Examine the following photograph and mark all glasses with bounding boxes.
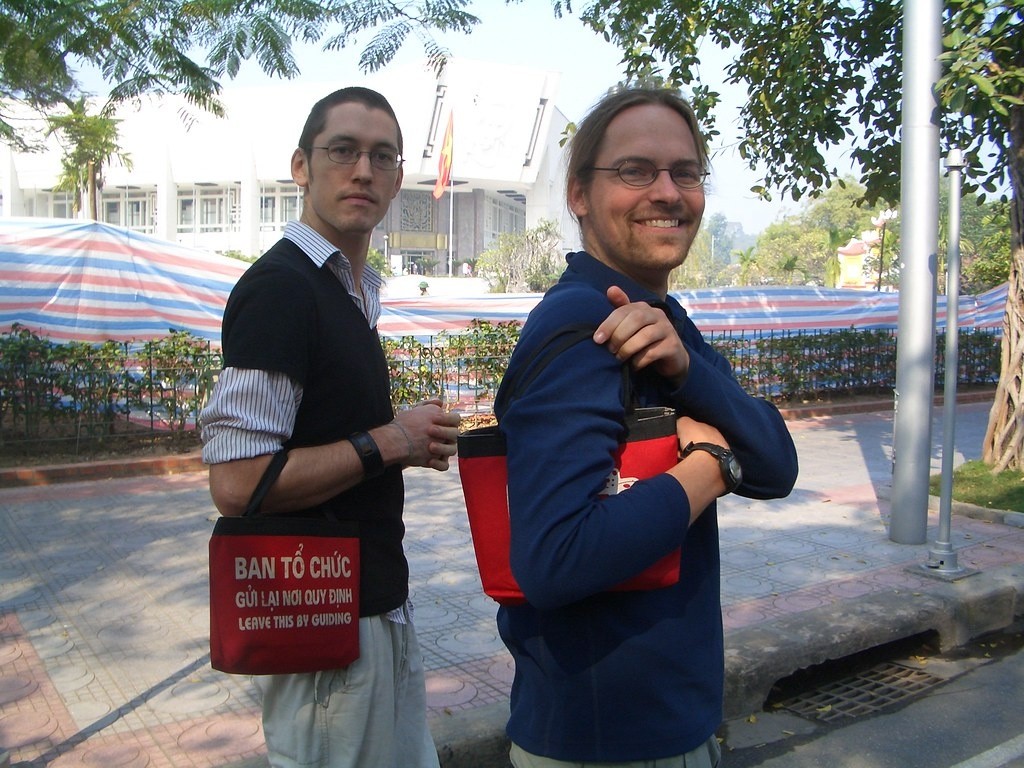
[302,144,405,171]
[584,158,711,189]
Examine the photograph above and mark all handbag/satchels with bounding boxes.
[458,322,681,608]
[210,447,361,675]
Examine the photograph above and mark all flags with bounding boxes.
[432,109,454,199]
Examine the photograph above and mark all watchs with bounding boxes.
[677,440,743,497]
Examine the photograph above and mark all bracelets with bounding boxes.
[349,429,383,489]
[389,421,412,468]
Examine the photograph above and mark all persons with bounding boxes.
[199,88,457,768]
[498,89,799,768]
[412,262,472,278]
